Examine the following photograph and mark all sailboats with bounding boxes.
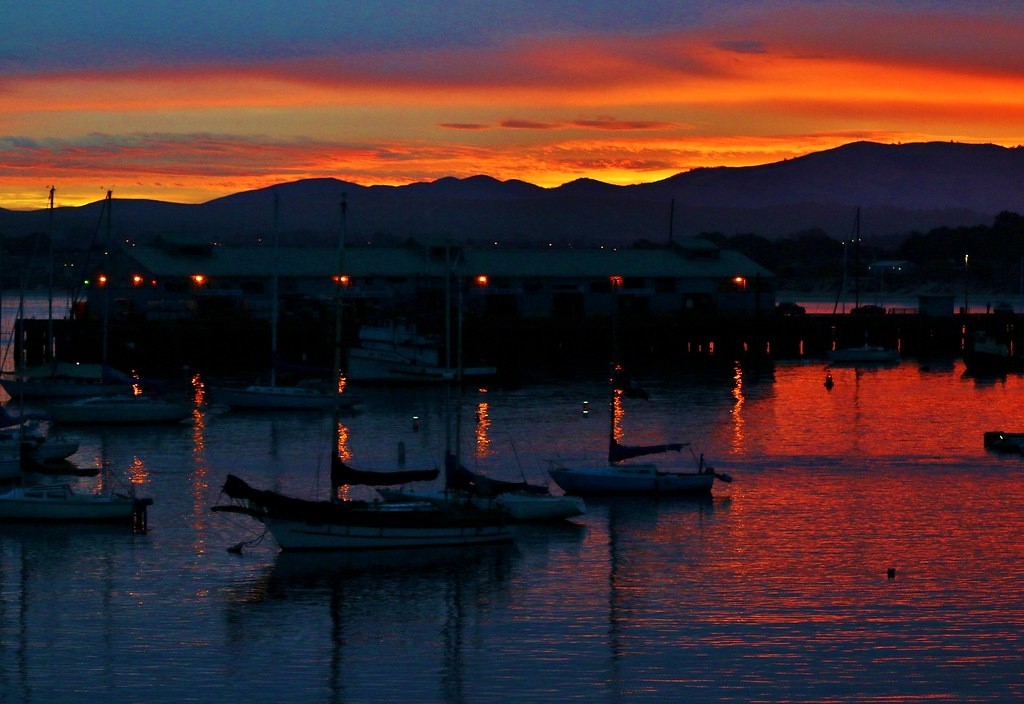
[1,162,1023,551]
